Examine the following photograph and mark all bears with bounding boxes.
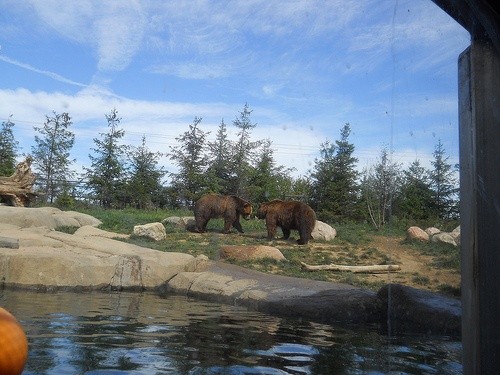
[194,193,253,233]
[255,198,316,246]
